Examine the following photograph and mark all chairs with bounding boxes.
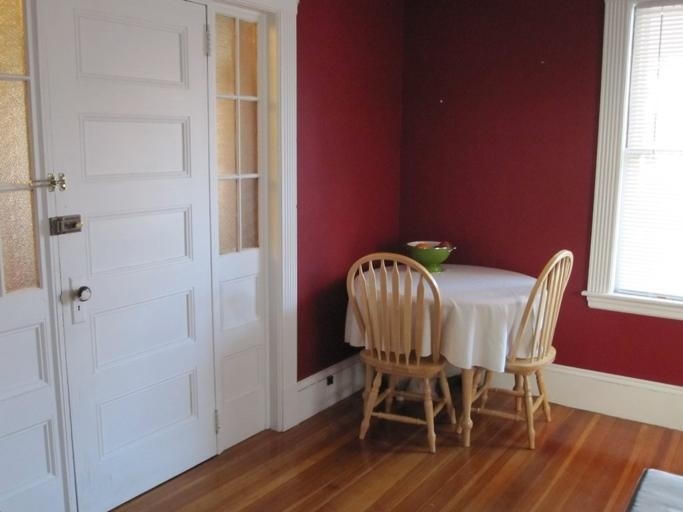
[347,253,456,453]
[457,249,574,450]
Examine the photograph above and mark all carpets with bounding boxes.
[630,467,683,512]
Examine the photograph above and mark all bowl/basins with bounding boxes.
[406,240,456,272]
